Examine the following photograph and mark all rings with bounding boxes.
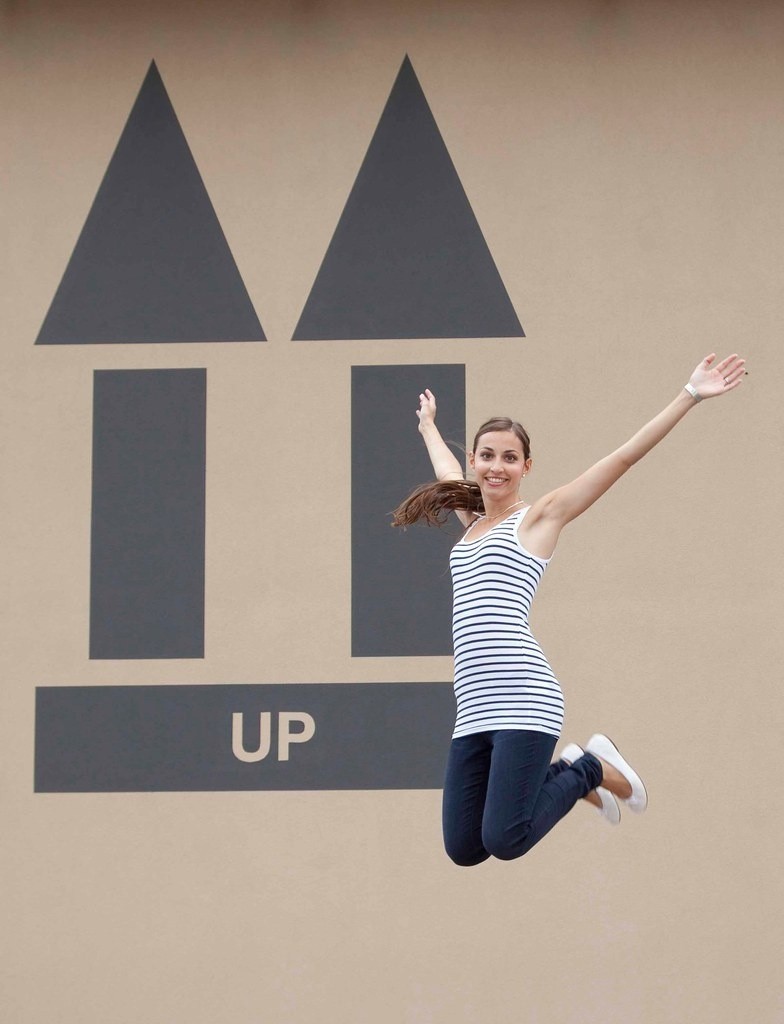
[724,377,730,383]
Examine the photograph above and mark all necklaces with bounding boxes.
[472,500,524,519]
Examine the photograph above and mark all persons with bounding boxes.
[390,353,745,867]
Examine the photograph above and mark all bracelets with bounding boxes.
[684,383,702,403]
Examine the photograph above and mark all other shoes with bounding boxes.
[560,742,621,823]
[586,733,648,814]
